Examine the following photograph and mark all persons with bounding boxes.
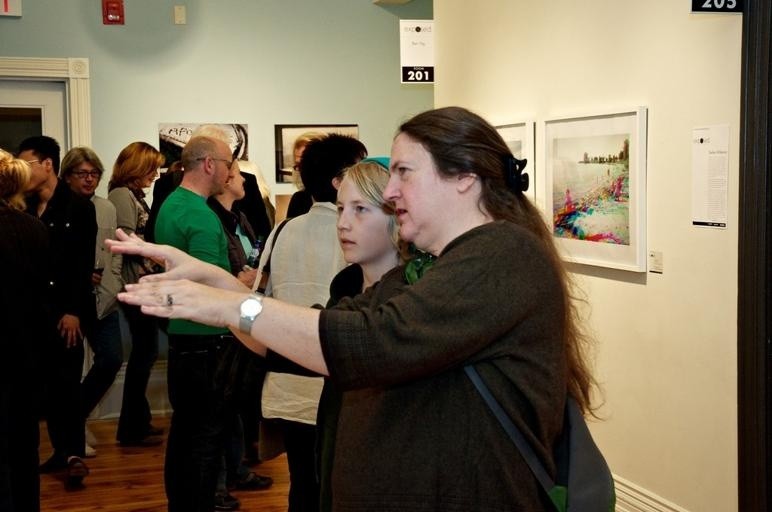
[1,135,438,512]
[105,107,606,511]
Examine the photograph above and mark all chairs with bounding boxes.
[274,125,359,184]
[489,114,535,201]
[532,105,647,275]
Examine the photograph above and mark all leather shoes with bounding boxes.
[228,469,273,490]
[147,423,164,436]
[120,433,161,448]
[63,455,90,480]
[39,456,60,473]
[215,488,241,509]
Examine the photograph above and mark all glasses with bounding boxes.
[28,156,46,167]
[196,155,234,170]
[75,168,102,179]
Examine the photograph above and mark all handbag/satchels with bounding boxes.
[465,358,616,511]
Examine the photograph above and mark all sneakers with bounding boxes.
[85,427,97,445]
[84,442,97,457]
